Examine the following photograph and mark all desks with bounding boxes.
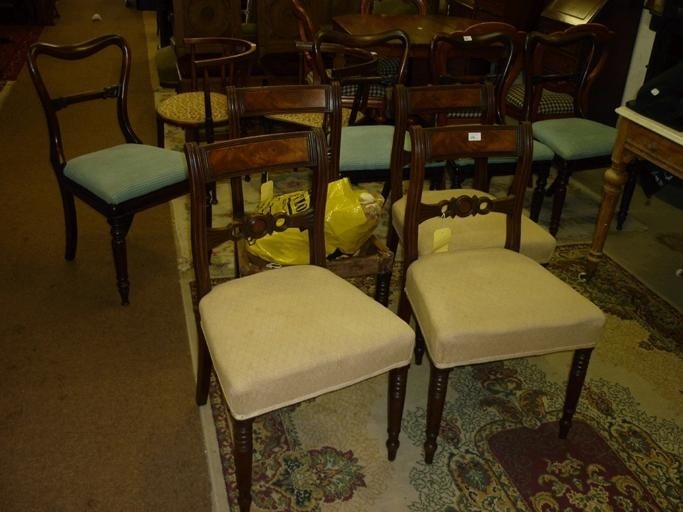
[583,104,682,283]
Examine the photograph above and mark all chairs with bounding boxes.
[386,81,555,270]
[26,35,188,304]
[181,128,416,511]
[522,23,633,239]
[156,2,639,151]
[393,120,606,463]
